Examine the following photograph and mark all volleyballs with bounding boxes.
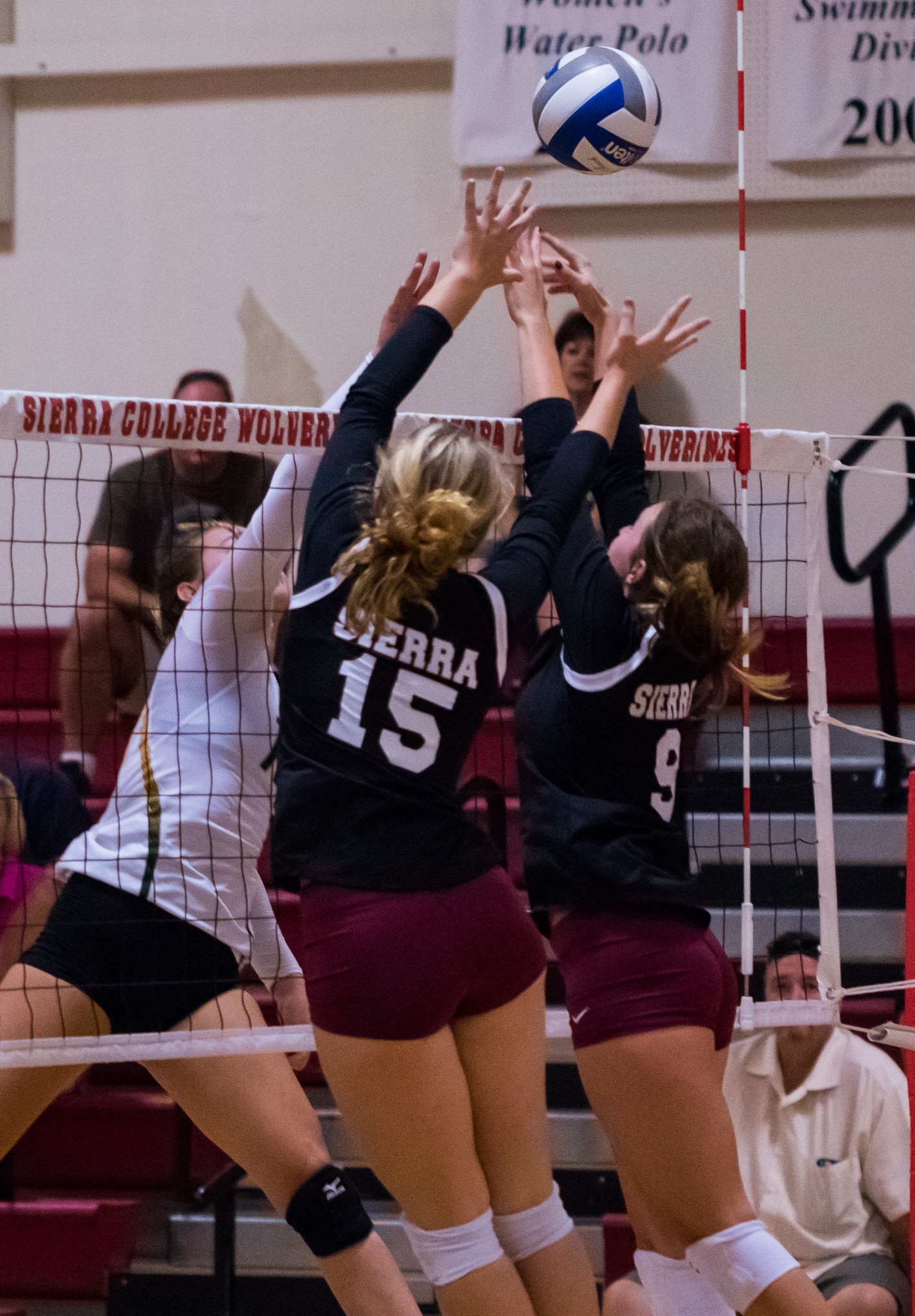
[532,45,663,177]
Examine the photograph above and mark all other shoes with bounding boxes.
[58,758,94,801]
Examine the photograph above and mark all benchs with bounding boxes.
[0,615,915,1316]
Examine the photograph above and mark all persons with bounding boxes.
[600,929,912,1316]
[509,312,654,693]
[1,166,915,1316]
[43,371,278,799]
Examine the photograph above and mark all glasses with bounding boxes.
[651,497,681,582]
[767,929,821,956]
